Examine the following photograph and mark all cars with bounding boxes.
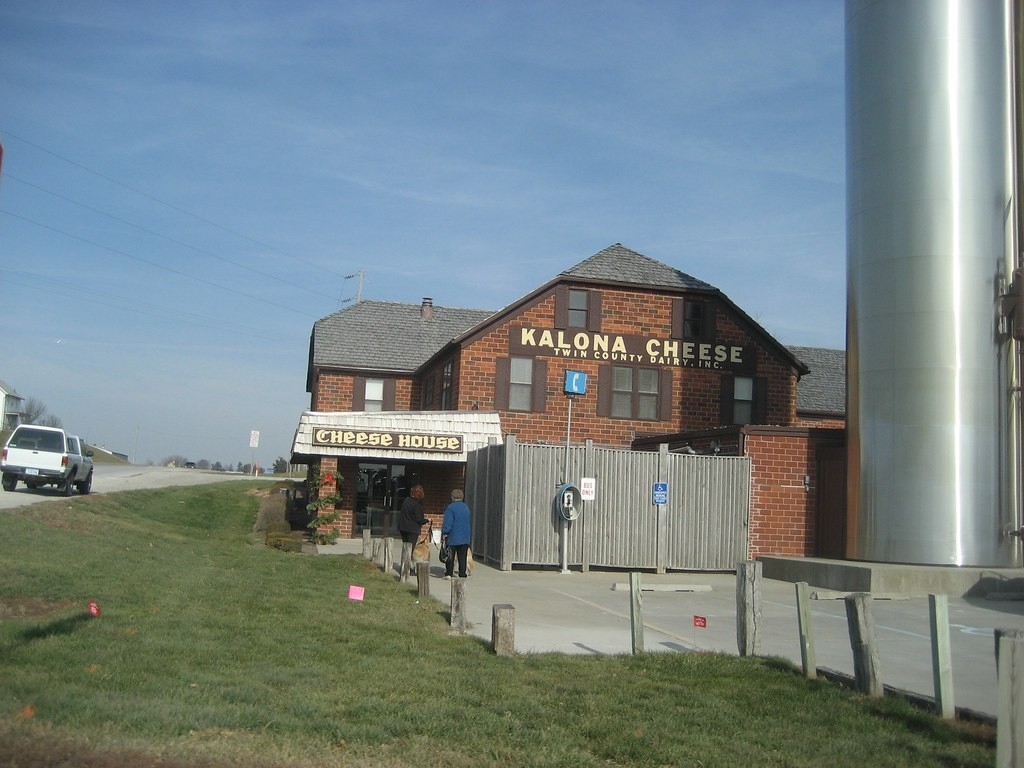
[184,462,196,470]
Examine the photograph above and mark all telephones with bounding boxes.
[564,491,573,507]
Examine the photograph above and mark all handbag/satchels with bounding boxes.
[439,538,451,563]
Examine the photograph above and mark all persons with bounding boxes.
[442,489,470,580]
[398,485,432,576]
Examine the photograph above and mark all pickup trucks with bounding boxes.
[0,424,94,497]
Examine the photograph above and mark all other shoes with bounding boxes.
[459,572,467,578]
[445,572,453,577]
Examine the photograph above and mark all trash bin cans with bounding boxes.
[280,487,296,507]
